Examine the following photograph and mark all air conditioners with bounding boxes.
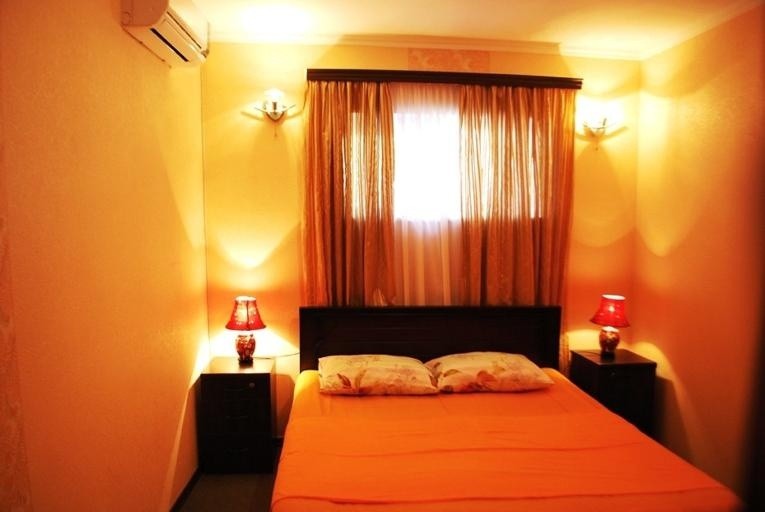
[120,0,211,69]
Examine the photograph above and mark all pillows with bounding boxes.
[318,355,438,395]
[425,353,556,392]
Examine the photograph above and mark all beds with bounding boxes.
[270,306,740,512]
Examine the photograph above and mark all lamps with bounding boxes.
[225,297,266,365]
[257,91,298,138]
[590,295,630,358]
[583,103,624,151]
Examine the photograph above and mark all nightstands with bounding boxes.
[201,359,271,476]
[571,350,657,444]
